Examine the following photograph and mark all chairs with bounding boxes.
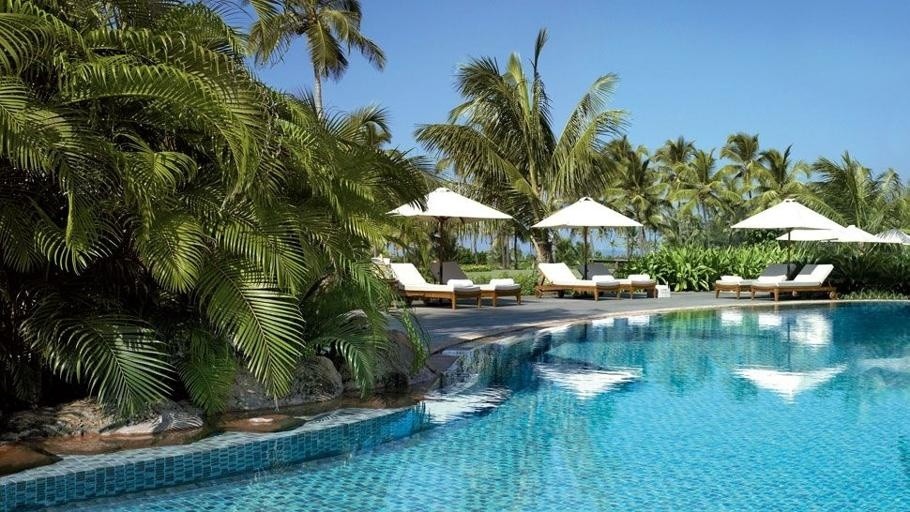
[707,262,840,303]
[389,258,657,310]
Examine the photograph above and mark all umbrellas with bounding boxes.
[530,195,645,279]
[729,197,910,280]
[380,188,515,284]
